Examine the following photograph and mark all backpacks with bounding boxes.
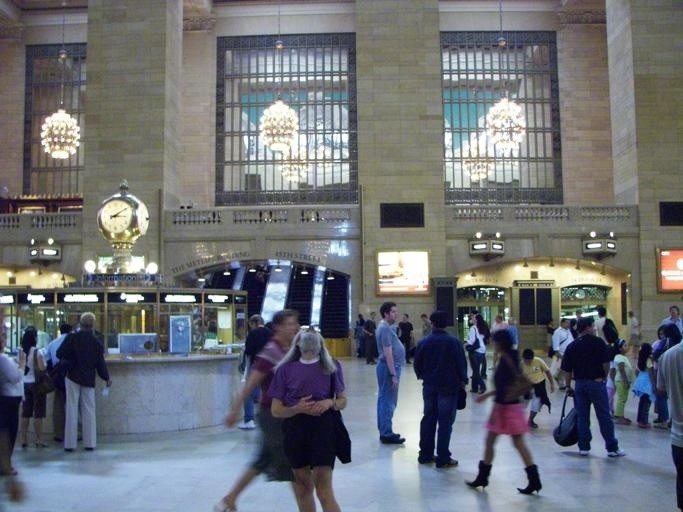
[553,390,579,446]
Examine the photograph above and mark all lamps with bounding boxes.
[280,146,310,183]
[41,0,81,160]
[471,257,607,276]
[197,259,335,282]
[258,1,299,152]
[28,237,62,267]
[470,231,505,261]
[582,231,618,260]
[486,0,527,151]
[463,137,498,182]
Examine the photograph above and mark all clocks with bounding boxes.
[100,198,134,233]
[137,202,149,235]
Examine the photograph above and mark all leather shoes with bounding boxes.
[469,374,487,394]
[419,455,458,467]
[21,436,95,451]
[379,433,405,444]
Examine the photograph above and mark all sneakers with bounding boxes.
[607,449,625,457]
[237,420,255,429]
[614,416,670,428]
[579,450,589,454]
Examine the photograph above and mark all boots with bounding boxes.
[467,461,492,488]
[528,411,538,428]
[517,464,542,494]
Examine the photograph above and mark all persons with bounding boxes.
[57,312,112,453]
[19,326,51,448]
[46,321,79,441]
[356,312,429,364]
[465,327,543,496]
[239,312,266,429]
[1,326,28,476]
[559,316,627,457]
[218,310,301,512]
[375,301,407,445]
[463,311,519,394]
[519,304,683,427]
[656,337,682,508]
[267,325,351,511]
[413,311,467,467]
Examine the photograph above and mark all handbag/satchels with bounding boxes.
[327,407,351,464]
[457,389,466,409]
[35,369,55,394]
[549,347,562,377]
[602,318,618,343]
[501,375,529,403]
[464,339,480,352]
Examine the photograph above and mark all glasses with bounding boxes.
[301,324,320,333]
